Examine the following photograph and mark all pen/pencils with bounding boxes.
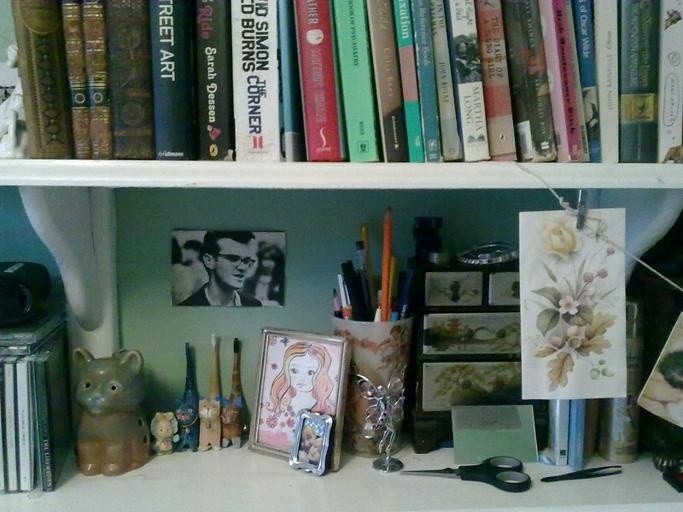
[333,205,415,322]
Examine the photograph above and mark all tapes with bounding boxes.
[429,252,450,264]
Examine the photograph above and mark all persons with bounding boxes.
[299,424,314,461]
[262,342,333,430]
[171,229,284,309]
[307,438,323,465]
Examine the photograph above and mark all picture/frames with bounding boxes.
[248,328,350,472]
[288,409,332,476]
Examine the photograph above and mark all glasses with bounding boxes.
[213,253,253,269]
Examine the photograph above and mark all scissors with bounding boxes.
[401,456,531,493]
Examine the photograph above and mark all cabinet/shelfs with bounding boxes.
[405,257,551,453]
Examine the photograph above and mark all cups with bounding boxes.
[332,309,414,458]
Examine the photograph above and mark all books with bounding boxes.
[552,396,570,467]
[0,317,72,496]
[9,1,683,160]
[602,302,645,467]
[569,396,586,473]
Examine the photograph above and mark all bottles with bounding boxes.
[597,302,640,465]
